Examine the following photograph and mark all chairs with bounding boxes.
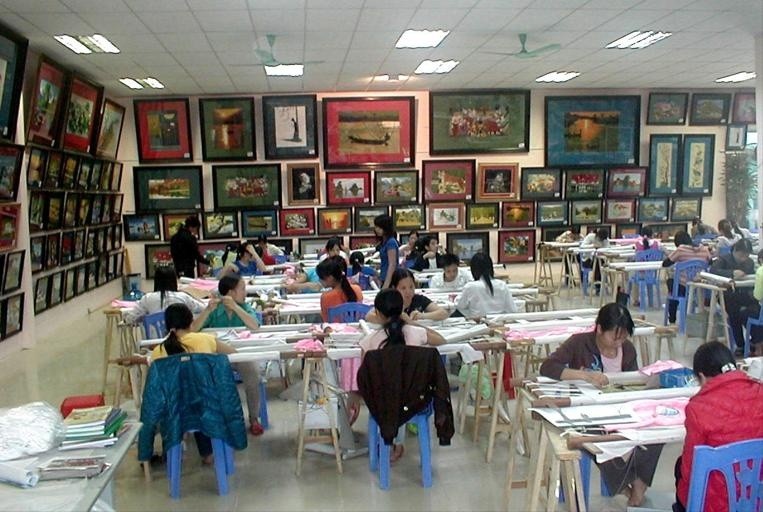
[356,345,455,490]
[138,352,248,499]
[686,439,762,512]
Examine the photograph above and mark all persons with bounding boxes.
[291,117,299,138]
[671,342,763,512]
[295,172,312,194]
[553,216,763,356]
[487,171,507,193]
[538,303,665,512]
[150,303,239,465]
[123,214,516,340]
[360,288,445,462]
[192,274,263,436]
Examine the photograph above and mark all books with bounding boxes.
[37,454,106,480]
[57,404,129,451]
[98,463,112,476]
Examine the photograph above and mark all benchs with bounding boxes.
[296,357,344,478]
[103,308,153,485]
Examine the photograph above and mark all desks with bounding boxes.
[1,420,142,512]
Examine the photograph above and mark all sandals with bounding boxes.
[250,421,262,434]
